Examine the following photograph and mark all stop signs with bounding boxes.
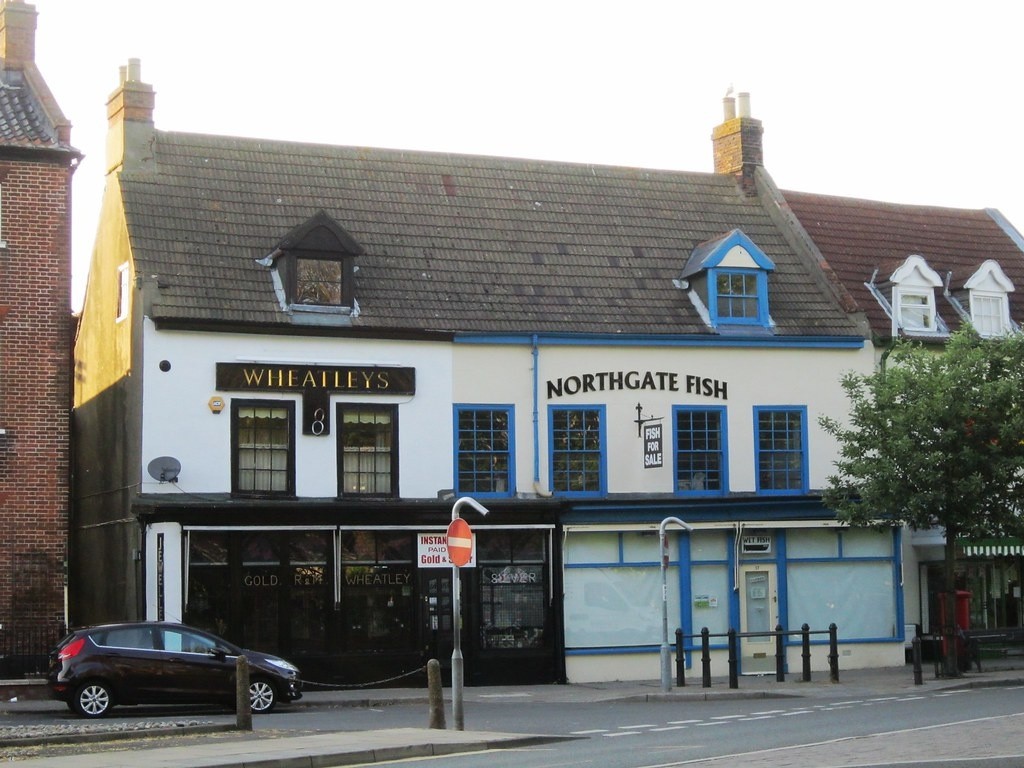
[662,534,669,570]
[445,518,474,566]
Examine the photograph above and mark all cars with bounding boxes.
[45,620,304,715]
